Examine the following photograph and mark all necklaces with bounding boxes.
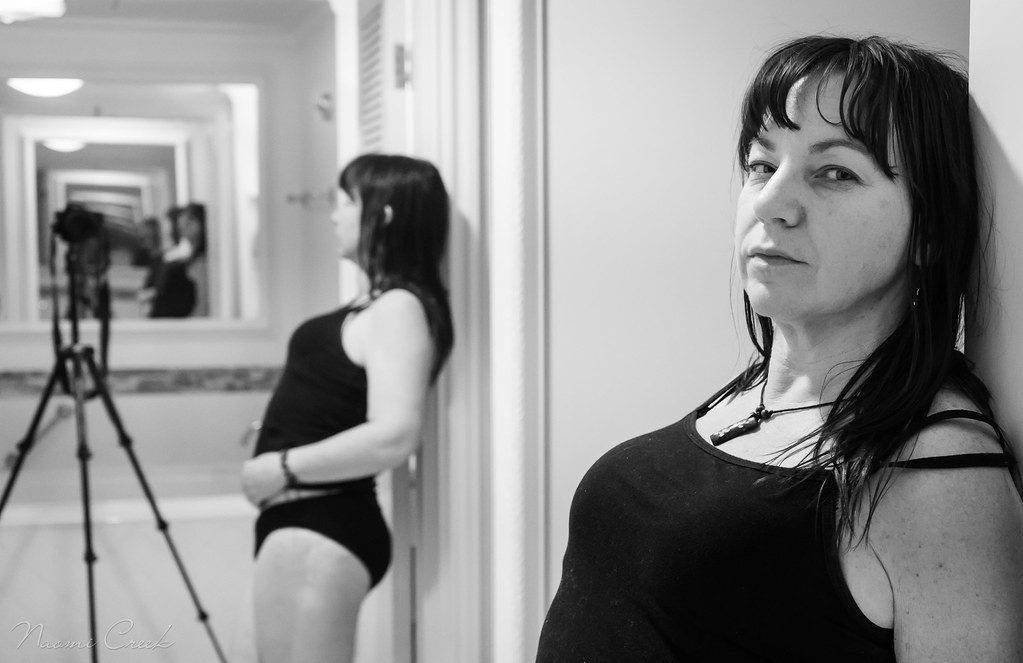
[710,378,877,448]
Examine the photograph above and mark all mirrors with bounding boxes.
[0,0,494,663]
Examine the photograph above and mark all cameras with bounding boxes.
[55,203,147,258]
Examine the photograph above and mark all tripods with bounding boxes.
[2,275,232,663]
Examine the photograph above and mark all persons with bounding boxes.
[532,35,1020,662]
[131,201,208,318]
[243,153,456,663]
